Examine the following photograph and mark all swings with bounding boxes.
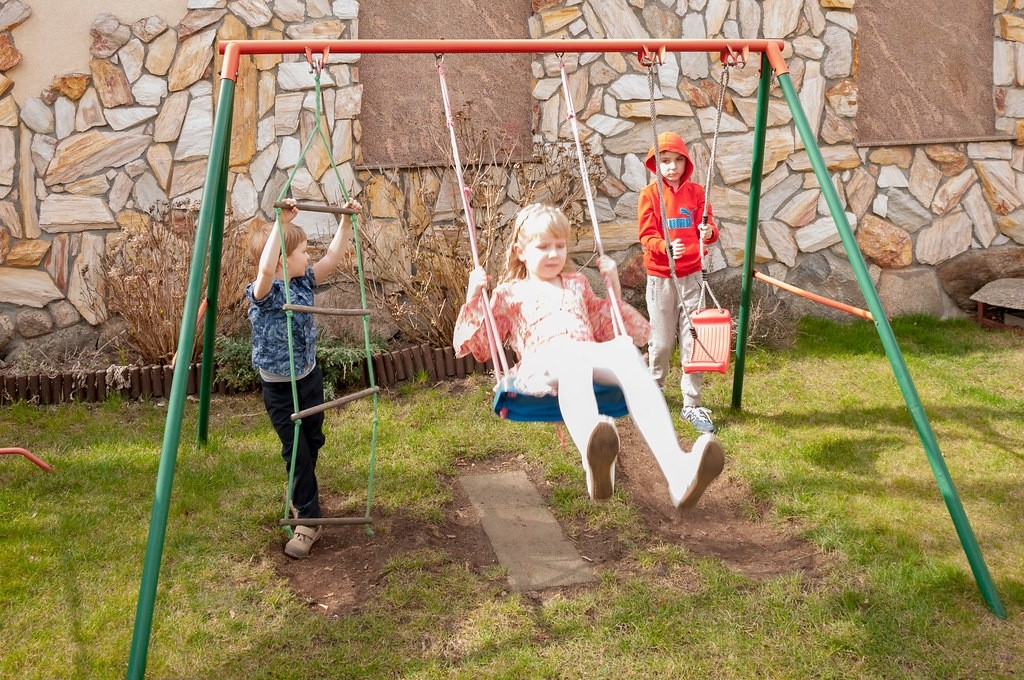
[432,50,637,427]
[639,51,743,374]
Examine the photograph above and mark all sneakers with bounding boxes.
[679,405,717,435]
[289,501,298,520]
[285,525,323,558]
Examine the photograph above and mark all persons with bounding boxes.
[246,197,363,560]
[453,202,724,510]
[638,131,719,433]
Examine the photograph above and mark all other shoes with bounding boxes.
[669,435,725,511]
[586,417,620,500]
